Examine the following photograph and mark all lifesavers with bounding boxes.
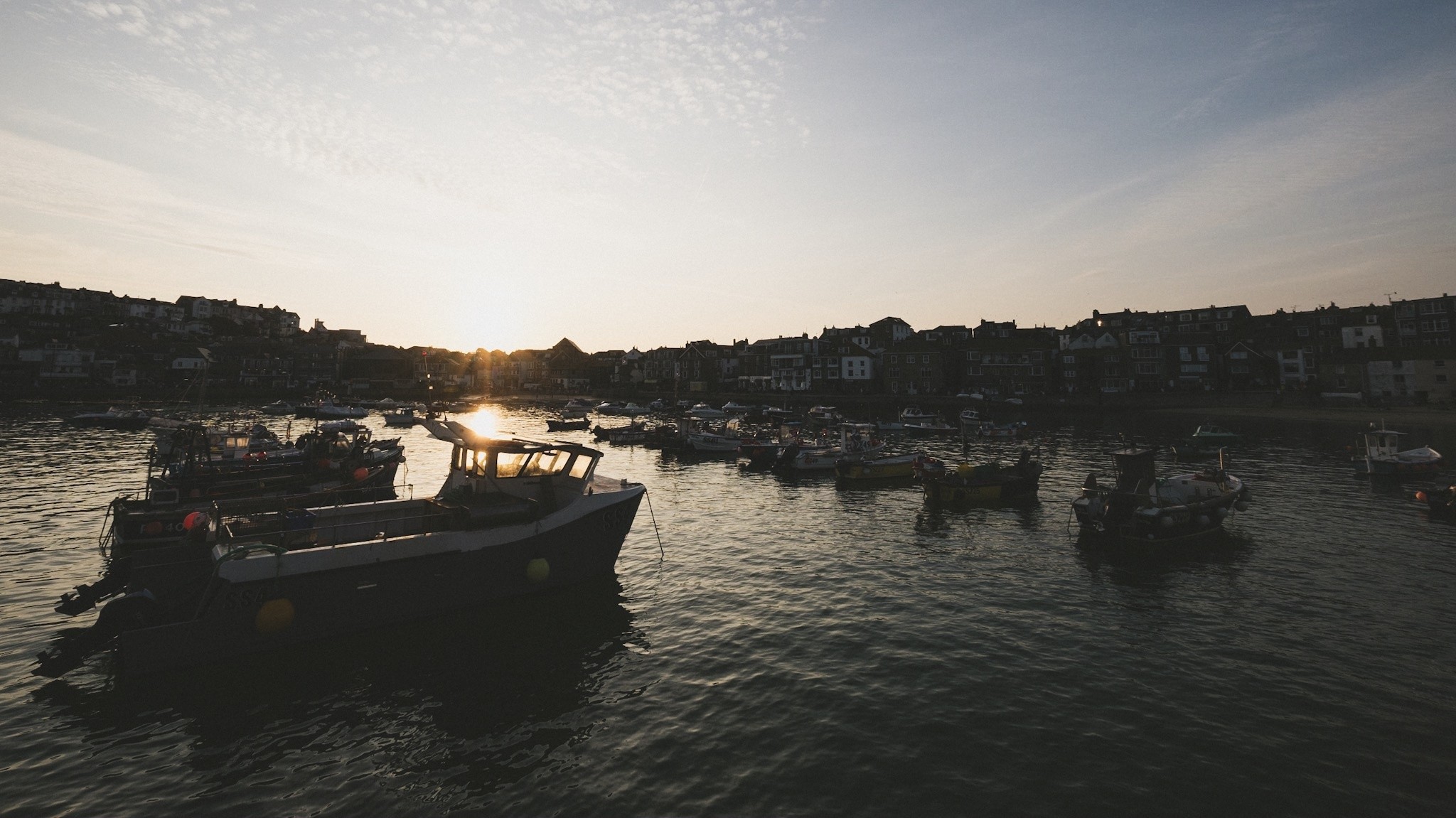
[243,452,267,460]
[148,520,165,536]
[184,512,208,529]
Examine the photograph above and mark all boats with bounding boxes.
[1351,418,1456,510]
[394,399,468,418]
[912,414,1042,510]
[1071,426,1244,559]
[6,399,84,416]
[31,348,405,679]
[544,397,1030,480]
[203,423,647,669]
